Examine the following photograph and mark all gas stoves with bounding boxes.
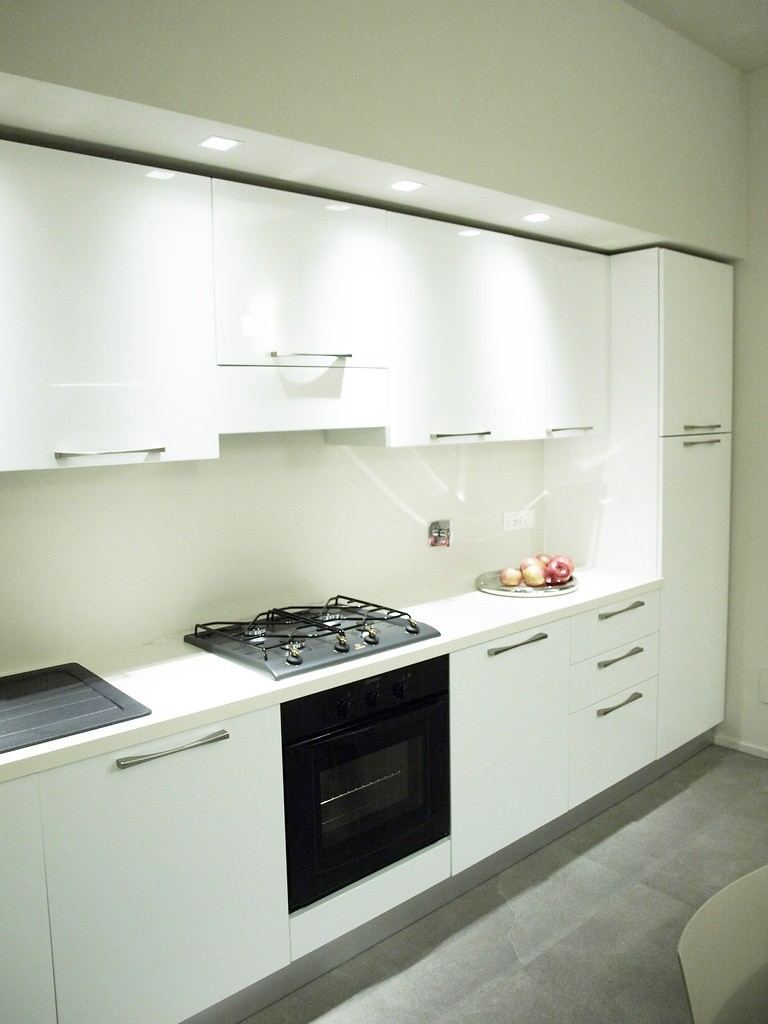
[183,597,442,679]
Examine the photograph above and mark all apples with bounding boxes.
[499,554,573,586]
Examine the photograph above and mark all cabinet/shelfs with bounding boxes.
[611,433,732,760]
[549,240,611,440]
[611,248,733,437]
[0,775,57,1024]
[212,177,387,437]
[40,704,291,1024]
[571,587,659,813]
[388,210,549,451]
[451,617,572,874]
[0,141,220,476]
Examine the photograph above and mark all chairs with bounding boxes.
[677,865,768,1024]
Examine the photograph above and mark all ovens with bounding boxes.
[279,653,470,914]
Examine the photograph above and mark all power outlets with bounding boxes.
[519,511,535,528]
[504,513,520,531]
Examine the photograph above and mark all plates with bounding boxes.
[475,570,579,597]
